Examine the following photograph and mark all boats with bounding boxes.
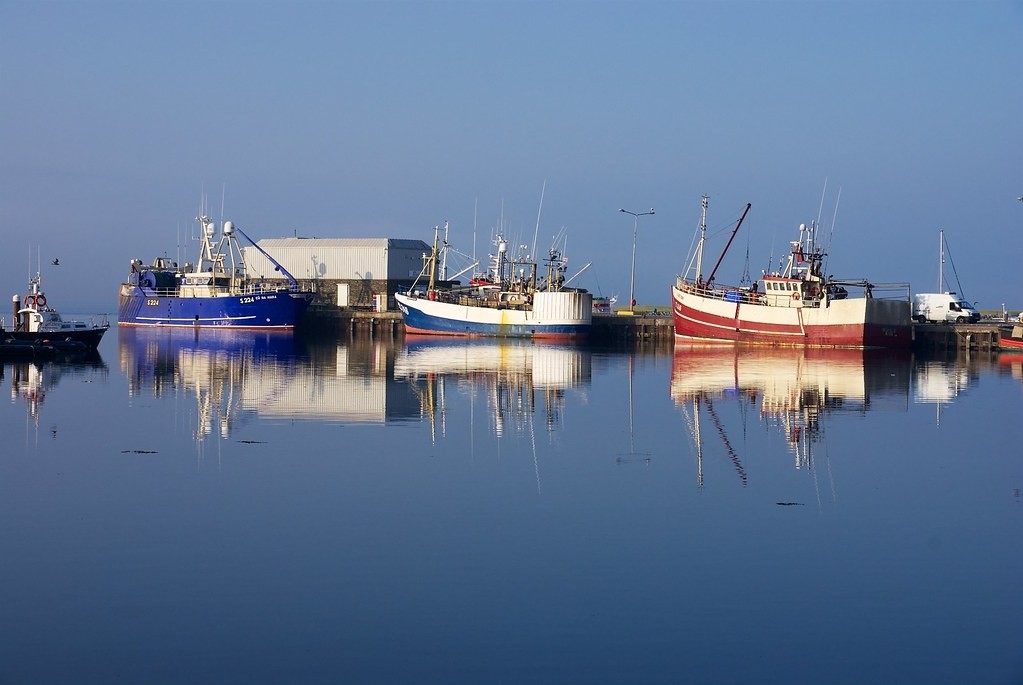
[0,358,59,448]
[670,193,911,348]
[117,181,315,333]
[672,349,909,509]
[999,339,1023,351]
[395,181,592,337]
[0,243,108,353]
[115,329,305,469]
[394,336,593,497]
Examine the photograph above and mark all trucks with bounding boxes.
[911,293,981,324]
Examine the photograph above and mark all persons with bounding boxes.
[697,274,703,291]
[752,281,758,293]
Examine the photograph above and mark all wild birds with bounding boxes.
[50,258,60,266]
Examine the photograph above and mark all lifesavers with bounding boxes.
[527,295,532,302]
[793,292,800,301]
[510,296,517,301]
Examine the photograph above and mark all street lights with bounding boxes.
[612,356,653,467]
[618,209,656,310]
